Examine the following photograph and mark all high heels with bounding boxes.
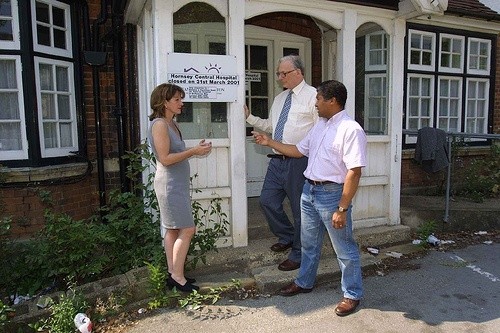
[165,271,196,286]
[167,277,199,293]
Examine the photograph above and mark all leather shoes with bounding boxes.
[280,282,312,296]
[335,298,359,315]
[271,242,293,252]
[278,259,300,271]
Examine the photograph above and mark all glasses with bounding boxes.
[276,69,297,78]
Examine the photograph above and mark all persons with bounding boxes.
[251,80,368,316]
[147,84,211,292]
[243,55,323,271]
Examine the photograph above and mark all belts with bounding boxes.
[267,154,292,159]
[307,179,335,185]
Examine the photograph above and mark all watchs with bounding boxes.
[337,206,348,213]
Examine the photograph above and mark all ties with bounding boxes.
[274,90,294,143]
